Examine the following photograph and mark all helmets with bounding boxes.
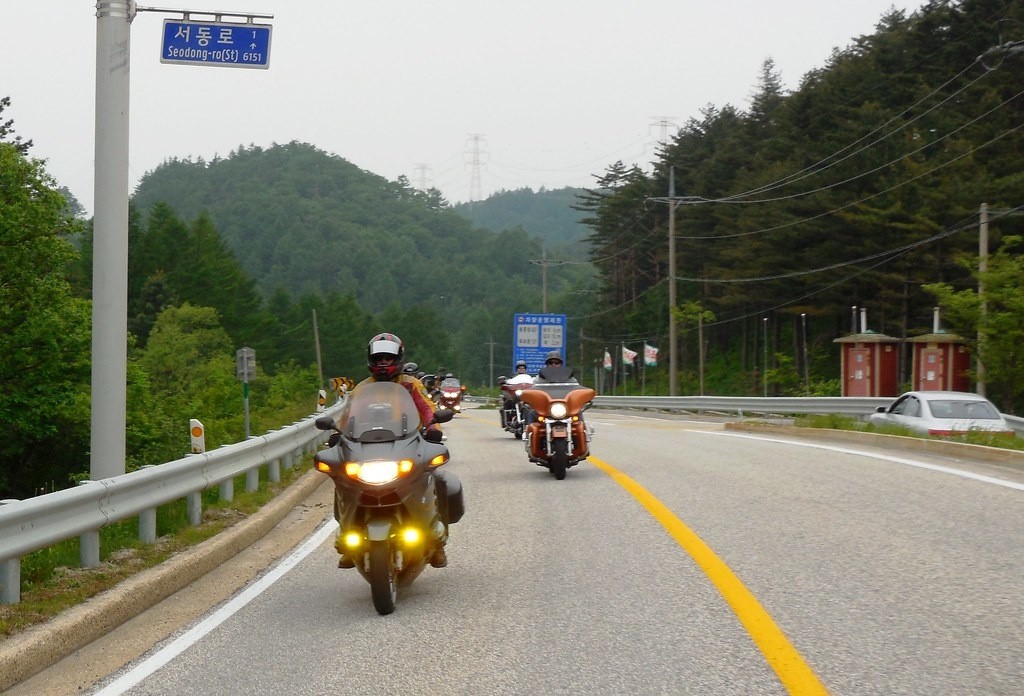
[515,360,526,369]
[545,351,563,364]
[368,333,403,380]
[417,372,426,378]
[404,362,418,378]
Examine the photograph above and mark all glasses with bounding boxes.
[374,354,395,361]
[546,360,560,364]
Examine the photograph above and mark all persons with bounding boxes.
[338,333,449,569]
[513,351,591,461]
[502,360,527,431]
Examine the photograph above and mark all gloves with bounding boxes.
[426,429,443,443]
[327,434,340,446]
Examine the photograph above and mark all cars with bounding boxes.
[869,391,1016,442]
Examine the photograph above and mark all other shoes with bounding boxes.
[431,548,446,567]
[338,553,354,568]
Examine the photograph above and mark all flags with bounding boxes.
[623,347,637,366]
[603,352,612,370]
[645,345,659,366]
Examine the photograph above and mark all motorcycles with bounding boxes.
[439,377,468,416]
[499,372,541,440]
[515,366,598,480]
[313,381,465,615]
